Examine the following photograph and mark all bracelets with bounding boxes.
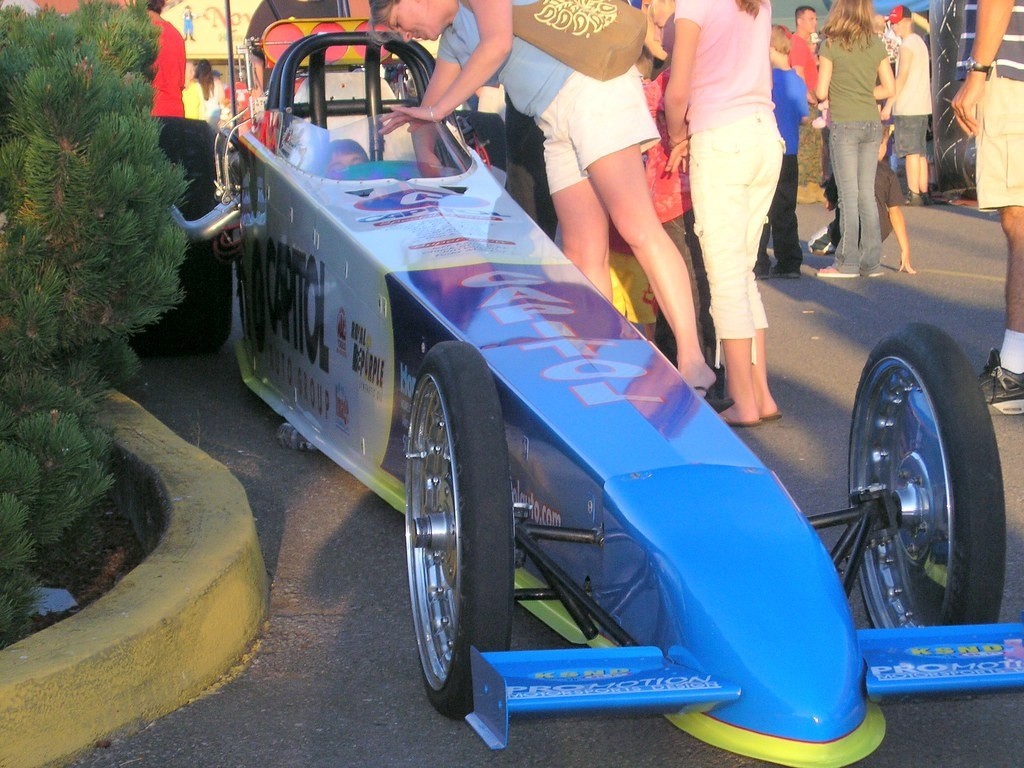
[429,105,439,123]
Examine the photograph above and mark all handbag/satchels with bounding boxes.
[461,0,647,83]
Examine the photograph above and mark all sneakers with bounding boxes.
[770,262,801,279]
[976,348,1024,416]
[752,260,772,280]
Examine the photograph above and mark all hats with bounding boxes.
[885,5,912,25]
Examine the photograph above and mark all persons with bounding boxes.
[129,1,933,397]
[663,0,787,427]
[950,0,1024,417]
[786,6,826,204]
[879,6,935,205]
[814,0,896,277]
[807,122,918,274]
[326,138,369,173]
[368,0,735,414]
[752,25,810,278]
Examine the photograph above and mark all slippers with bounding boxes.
[816,265,860,278]
[861,273,885,277]
[719,413,762,428]
[694,386,735,414]
[759,414,782,423]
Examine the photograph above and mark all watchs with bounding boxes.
[965,58,990,73]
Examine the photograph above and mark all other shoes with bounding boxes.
[904,196,935,206]
